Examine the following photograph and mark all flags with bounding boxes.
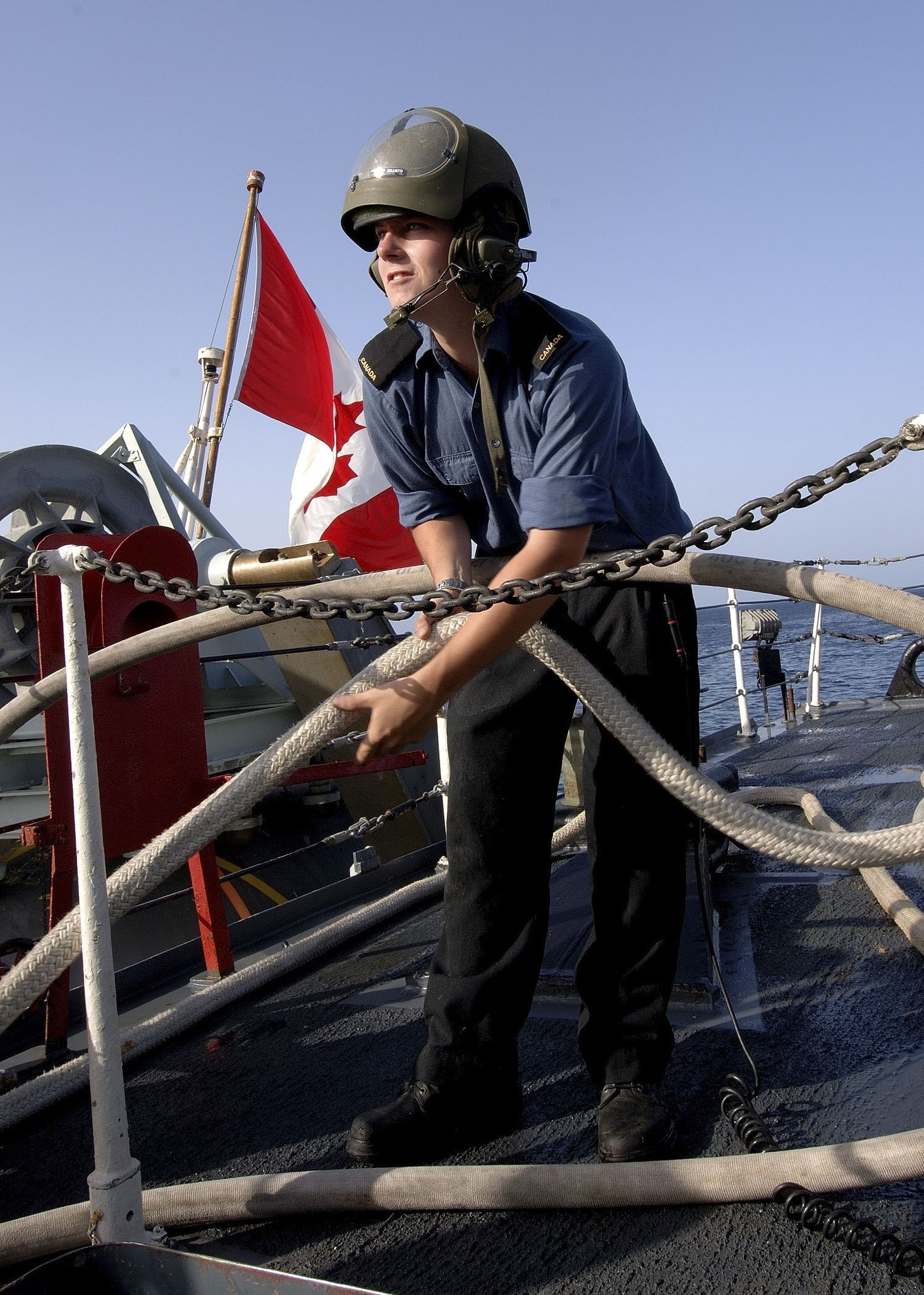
[233,206,424,577]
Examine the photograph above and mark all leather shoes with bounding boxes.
[597,1083,680,1164]
[345,1076,524,1161]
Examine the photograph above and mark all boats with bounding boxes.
[4,168,447,1043]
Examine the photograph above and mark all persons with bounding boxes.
[329,109,699,1161]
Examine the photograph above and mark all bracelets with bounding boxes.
[436,578,469,592]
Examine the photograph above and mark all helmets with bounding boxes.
[348,121,534,240]
